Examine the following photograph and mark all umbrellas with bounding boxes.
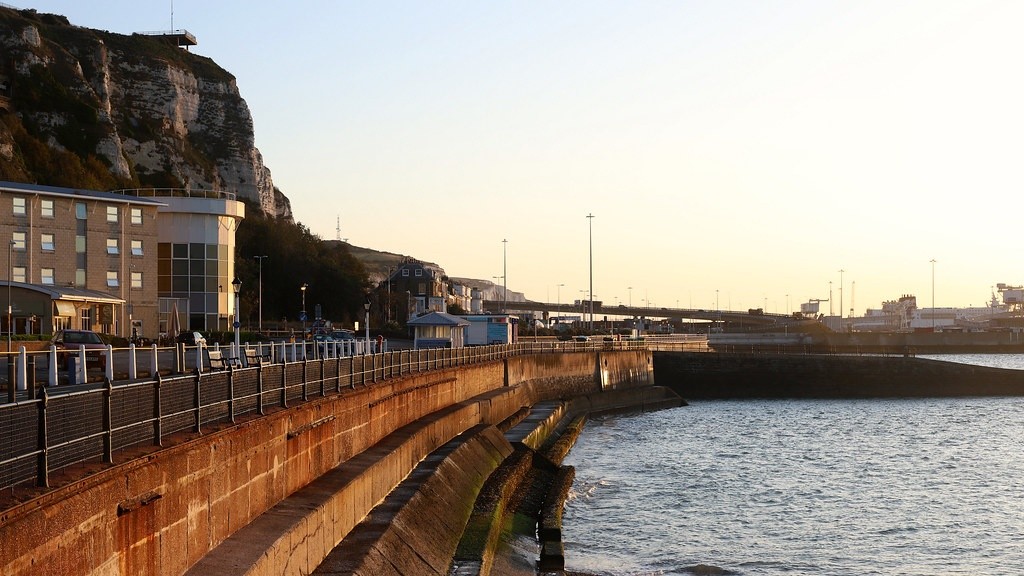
[168,303,180,347]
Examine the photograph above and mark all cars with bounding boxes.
[307,321,356,352]
[47,330,107,370]
[176,332,207,349]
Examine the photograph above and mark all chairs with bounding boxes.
[244,349,272,367]
[207,349,239,373]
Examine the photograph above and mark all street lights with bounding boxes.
[364,298,371,354]
[786,294,788,314]
[255,256,267,332]
[7,239,16,351]
[586,212,595,331]
[405,291,410,321]
[385,265,395,323]
[838,269,844,318]
[301,284,307,331]
[580,289,589,329]
[493,277,504,311]
[716,290,719,311]
[627,287,632,306]
[930,260,937,331]
[556,284,563,326]
[231,275,242,359]
[829,281,833,317]
[502,238,507,304]
